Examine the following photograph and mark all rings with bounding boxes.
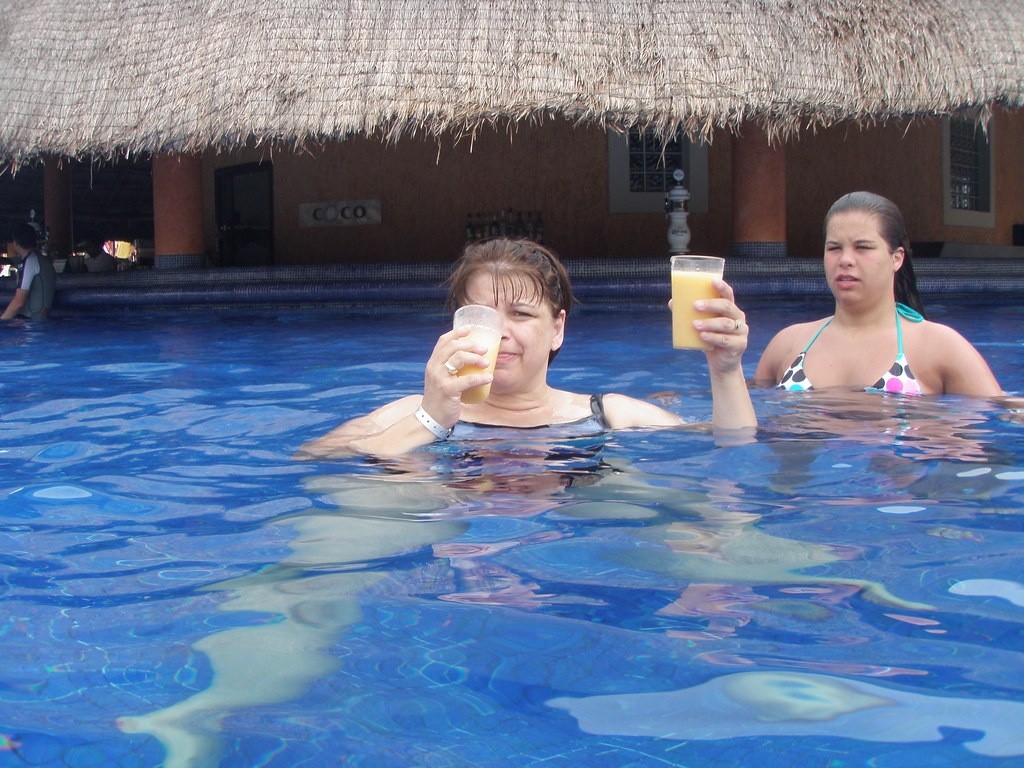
[732,318,740,331]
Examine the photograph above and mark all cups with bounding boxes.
[452,304,504,402]
[670,254,725,351]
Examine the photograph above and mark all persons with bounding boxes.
[753,191,1006,399]
[0,230,58,322]
[75,233,119,274]
[299,236,759,458]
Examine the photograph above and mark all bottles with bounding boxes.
[499,204,506,236]
[476,212,484,239]
[506,206,515,240]
[526,212,534,239]
[492,207,498,237]
[484,212,491,237]
[464,213,474,241]
[131,239,141,262]
[536,212,544,242]
[516,211,524,239]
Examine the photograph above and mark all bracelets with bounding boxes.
[414,406,452,440]
